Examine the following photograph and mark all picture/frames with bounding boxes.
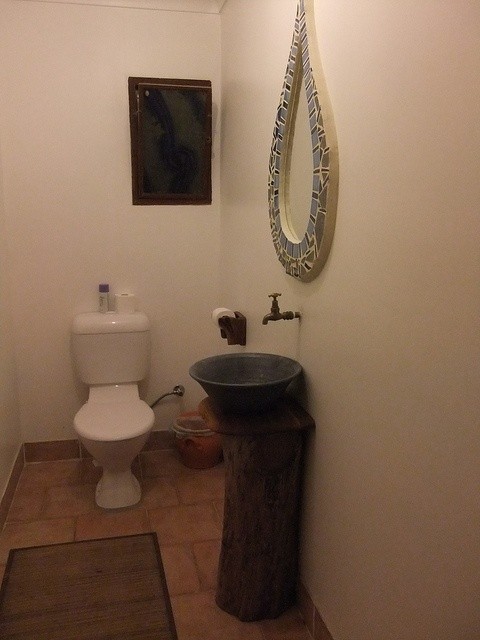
[128,77,212,206]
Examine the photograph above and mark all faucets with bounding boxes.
[262,292,300,326]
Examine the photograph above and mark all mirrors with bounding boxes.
[266,0,340,282]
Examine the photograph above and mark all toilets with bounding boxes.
[69,312,157,510]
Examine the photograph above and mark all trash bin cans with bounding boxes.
[169,415,223,470]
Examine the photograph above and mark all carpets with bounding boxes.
[1,532,179,640]
